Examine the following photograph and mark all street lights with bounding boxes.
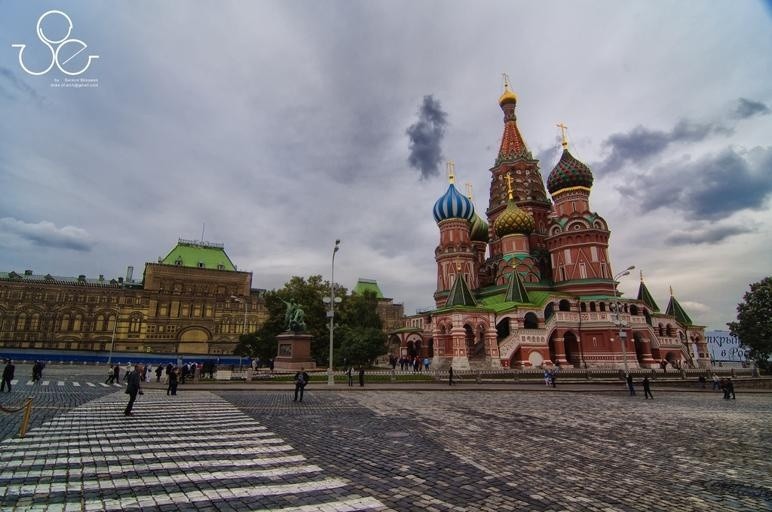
[230,294,248,339]
[610,263,641,380]
[325,238,345,386]
[103,303,123,365]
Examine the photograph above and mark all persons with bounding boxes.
[105,361,197,396]
[624,374,636,396]
[544,369,551,386]
[359,365,365,387]
[699,373,736,399]
[449,367,455,385]
[293,368,309,401]
[278,297,302,330]
[251,358,258,370]
[32,360,45,381]
[643,376,653,399]
[550,370,556,387]
[269,357,274,376]
[389,354,430,372]
[123,365,143,416]
[347,366,355,386]
[0,360,15,392]
